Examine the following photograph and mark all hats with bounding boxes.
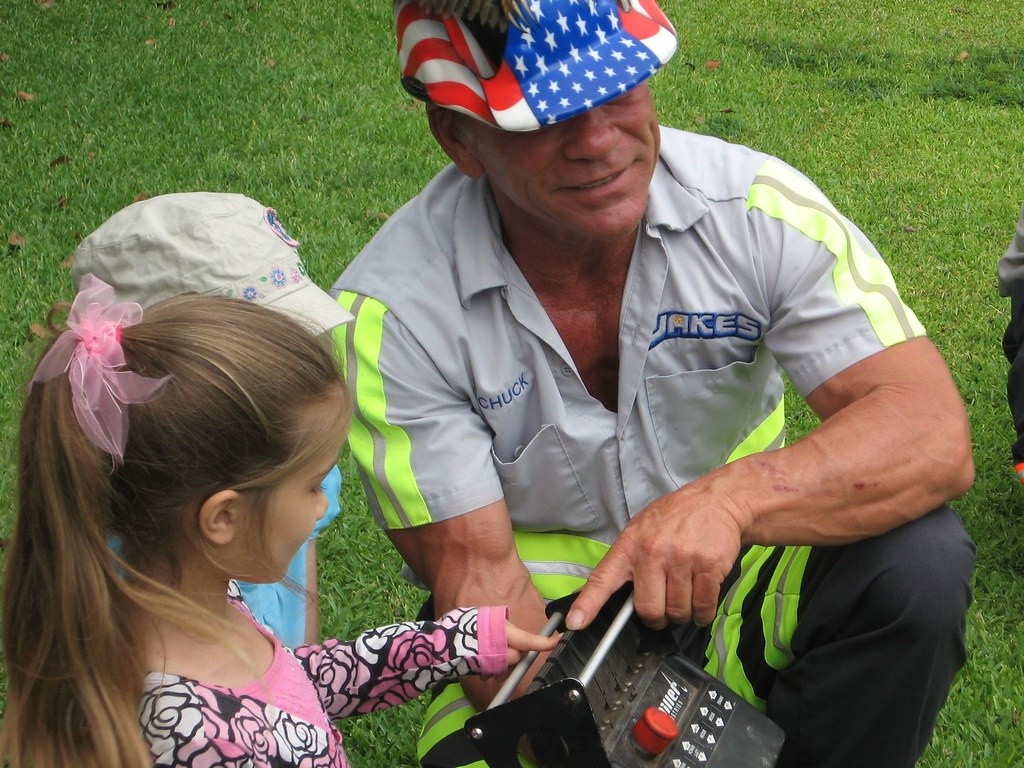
[74,189,357,342]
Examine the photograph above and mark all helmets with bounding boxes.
[394,0,681,131]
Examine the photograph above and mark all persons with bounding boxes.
[0,192,562,768]
[320,0,977,768]
[998,201,1024,485]
